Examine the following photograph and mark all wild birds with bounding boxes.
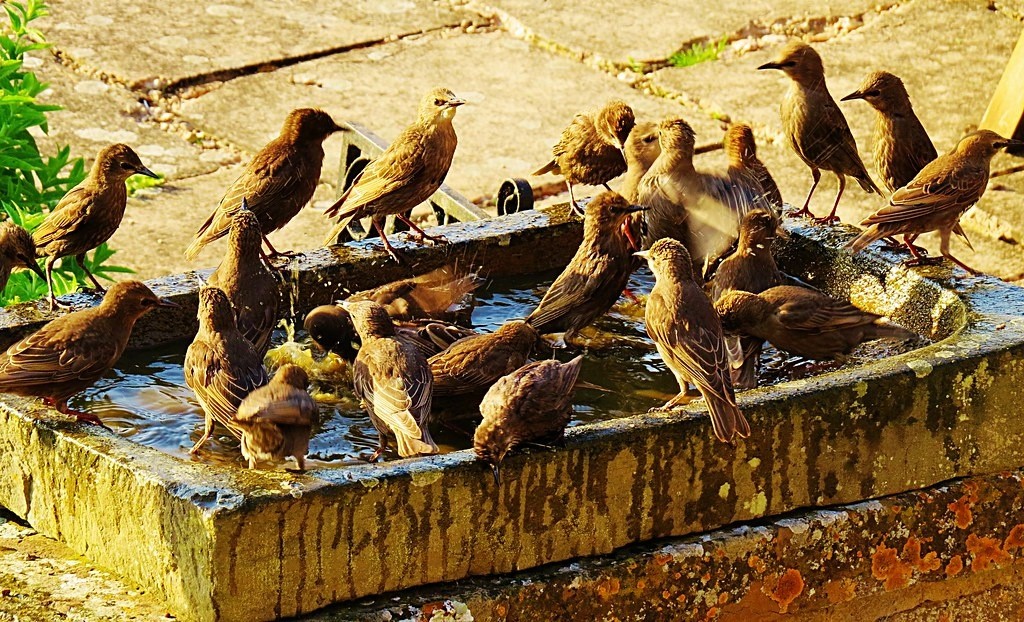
[0,40,1024,473]
